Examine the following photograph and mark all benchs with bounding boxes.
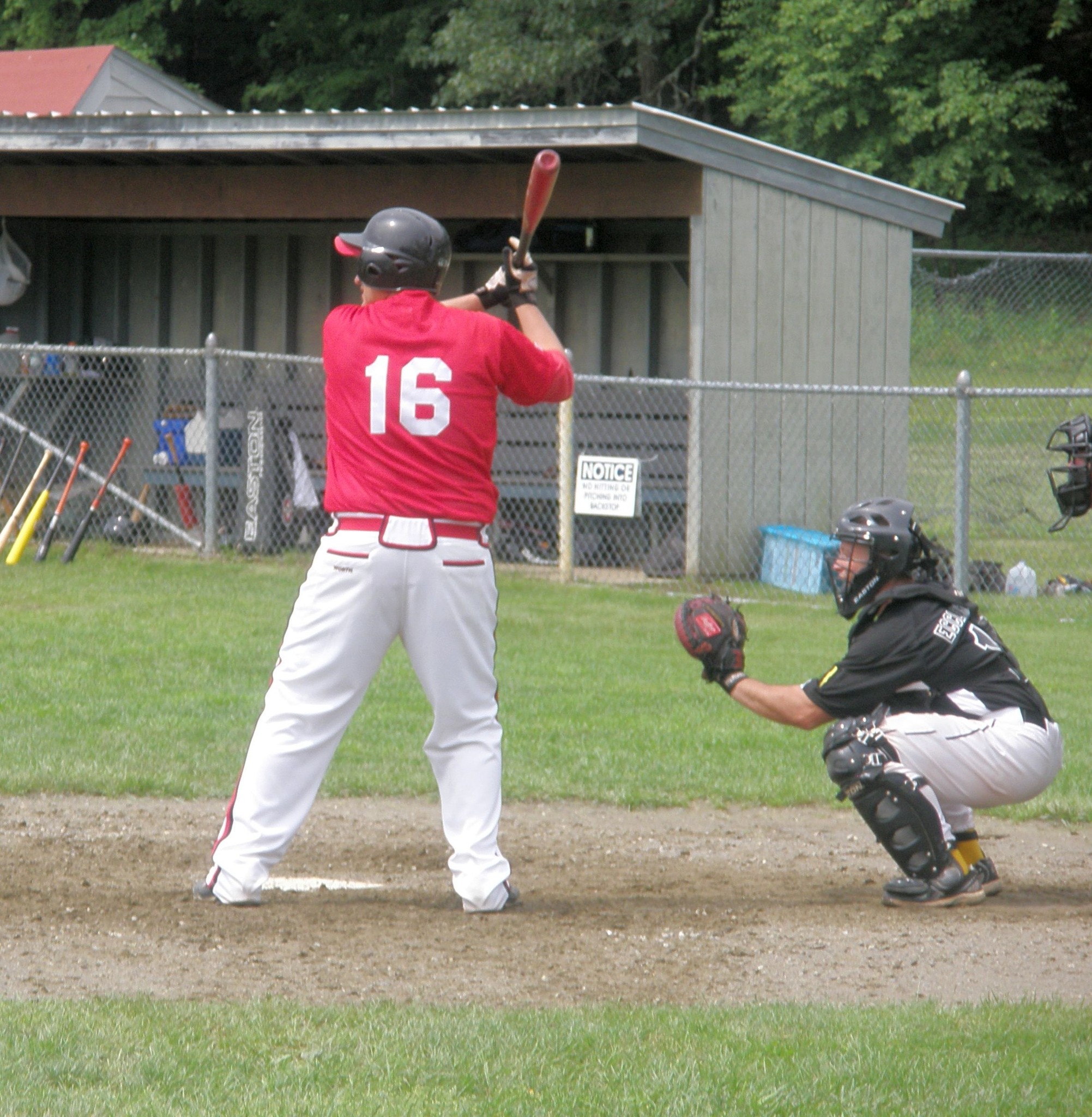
[144,384,689,563]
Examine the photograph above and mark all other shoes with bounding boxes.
[504,886,523,907]
[193,880,219,902]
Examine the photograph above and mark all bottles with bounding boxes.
[1007,561,1036,597]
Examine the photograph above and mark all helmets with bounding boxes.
[340,206,451,294]
[822,499,914,618]
[1046,413,1092,532]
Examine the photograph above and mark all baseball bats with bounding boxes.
[0,431,132,564]
[163,431,198,529]
[513,149,561,268]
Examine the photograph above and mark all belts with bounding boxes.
[1021,707,1047,731]
[337,518,479,540]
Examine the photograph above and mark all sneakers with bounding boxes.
[975,858,1002,894]
[882,866,986,908]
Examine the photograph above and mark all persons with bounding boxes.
[197,207,575,918]
[669,498,1063,907]
[1043,412,1092,533]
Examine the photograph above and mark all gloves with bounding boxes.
[503,236,539,307]
[473,263,509,309]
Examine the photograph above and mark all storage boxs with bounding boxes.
[753,525,840,592]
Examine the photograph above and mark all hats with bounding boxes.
[335,236,361,256]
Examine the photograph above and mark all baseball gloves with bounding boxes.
[674,592,748,683]
